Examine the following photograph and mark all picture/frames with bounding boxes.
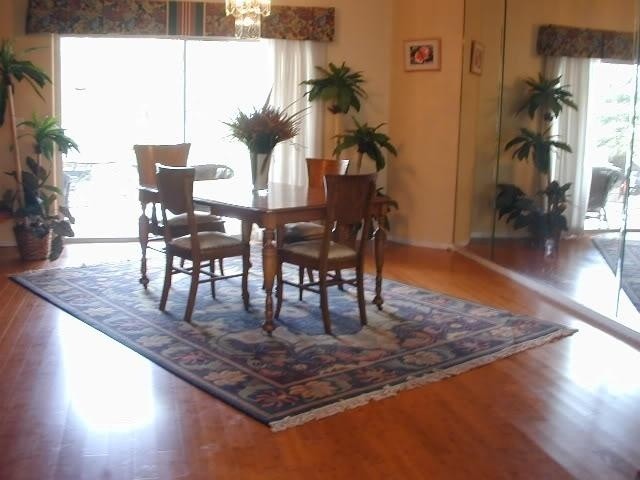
[466,38,486,76]
[401,35,445,73]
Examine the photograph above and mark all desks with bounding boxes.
[137,175,395,316]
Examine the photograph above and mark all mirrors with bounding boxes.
[452,0,639,341]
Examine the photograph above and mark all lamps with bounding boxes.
[222,0,272,42]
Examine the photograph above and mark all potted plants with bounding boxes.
[0,38,81,262]
[485,69,570,253]
[296,61,399,251]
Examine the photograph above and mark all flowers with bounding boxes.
[222,86,313,156]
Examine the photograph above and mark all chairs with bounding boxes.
[584,164,620,221]
[150,160,251,323]
[269,171,380,338]
[131,138,227,292]
[180,161,237,216]
[258,156,352,303]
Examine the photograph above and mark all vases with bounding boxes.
[250,151,271,193]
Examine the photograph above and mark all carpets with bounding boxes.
[587,235,638,315]
[4,240,581,436]
